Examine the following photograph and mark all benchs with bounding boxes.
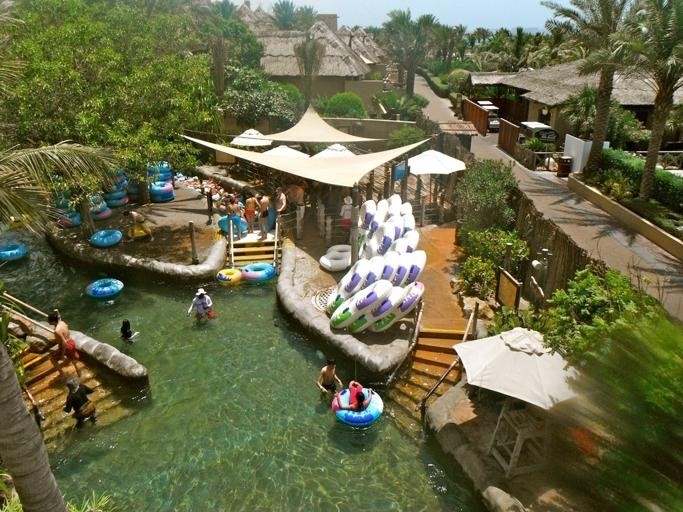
[333,218,353,241]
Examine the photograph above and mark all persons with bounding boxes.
[120,320,138,346]
[48,312,82,378]
[178,175,353,241]
[187,288,213,323]
[120,210,154,243]
[316,358,343,399]
[63,376,96,428]
[334,381,372,412]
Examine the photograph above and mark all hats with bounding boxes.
[343,196,352,204]
[47,311,59,321]
[67,380,77,390]
[196,288,207,296]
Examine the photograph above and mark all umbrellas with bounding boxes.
[396,149,466,207]
[452,327,579,411]
[230,129,356,159]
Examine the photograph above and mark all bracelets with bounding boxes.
[320,385,323,389]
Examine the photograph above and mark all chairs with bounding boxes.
[487,396,551,481]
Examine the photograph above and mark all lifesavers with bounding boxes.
[85,278,124,298]
[127,222,156,239]
[47,151,176,228]
[244,262,276,283]
[218,216,250,234]
[217,268,241,284]
[318,194,425,335]
[178,174,259,223]
[90,230,123,247]
[332,387,384,428]
[1,240,30,261]
[258,203,276,231]
